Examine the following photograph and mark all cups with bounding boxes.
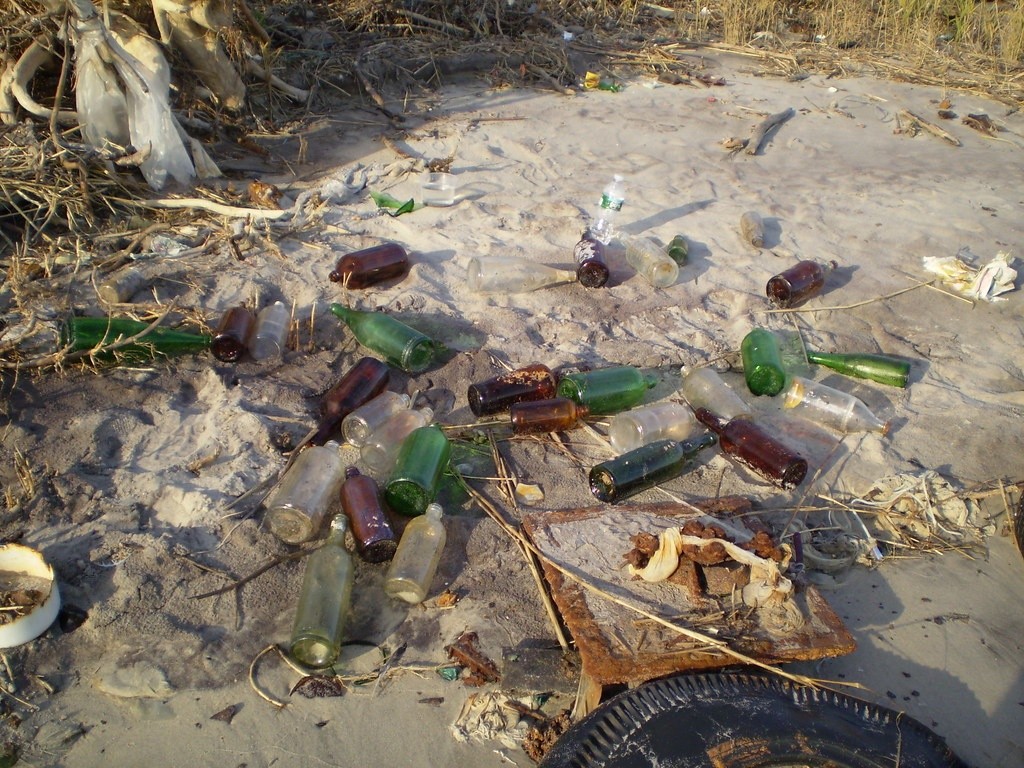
[419,172,457,206]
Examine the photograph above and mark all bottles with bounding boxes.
[329,244,410,290]
[680,365,753,425]
[766,259,837,309]
[740,211,765,247]
[574,228,609,287]
[590,173,625,246]
[618,231,679,289]
[694,406,808,491]
[667,235,688,266]
[466,258,577,292]
[742,325,785,397]
[808,351,910,387]
[784,375,889,436]
[67,302,717,668]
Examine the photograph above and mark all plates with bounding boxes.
[99,266,144,307]
